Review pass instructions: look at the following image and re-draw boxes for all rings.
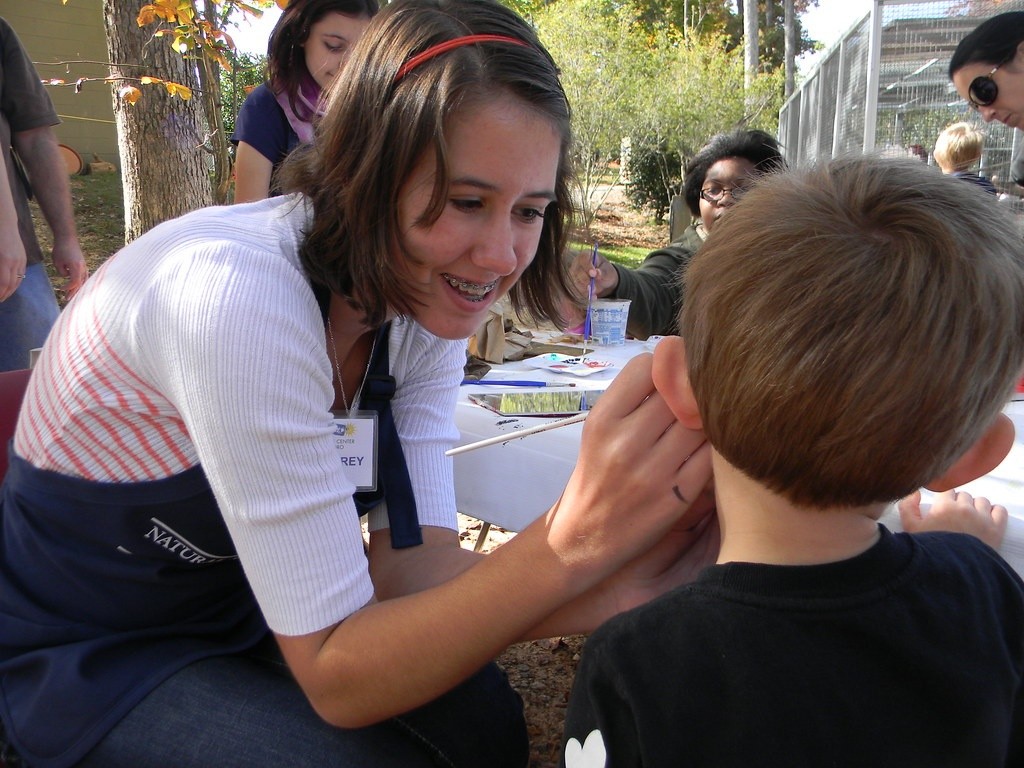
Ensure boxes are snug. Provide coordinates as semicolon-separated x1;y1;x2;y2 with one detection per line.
17;274;26;279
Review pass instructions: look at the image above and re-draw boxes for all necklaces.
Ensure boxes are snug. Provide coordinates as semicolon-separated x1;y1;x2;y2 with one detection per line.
326;317;379;418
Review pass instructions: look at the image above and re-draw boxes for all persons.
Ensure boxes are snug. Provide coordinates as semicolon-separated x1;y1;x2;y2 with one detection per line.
565;128;790;341
0;0;724;768
557;150;1024;768
933;122;997;198
0;16;89;372
229;0;379;205
0;144;27;304
947;11;1024;133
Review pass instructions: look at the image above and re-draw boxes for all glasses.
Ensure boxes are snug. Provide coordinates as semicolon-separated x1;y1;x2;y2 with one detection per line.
700;188;749;200
967;49;1015;112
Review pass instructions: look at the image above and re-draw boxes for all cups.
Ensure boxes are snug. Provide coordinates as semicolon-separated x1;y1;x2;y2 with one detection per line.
591;298;631;345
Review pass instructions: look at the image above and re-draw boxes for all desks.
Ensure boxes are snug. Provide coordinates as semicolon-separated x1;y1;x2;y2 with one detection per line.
453;328;662;553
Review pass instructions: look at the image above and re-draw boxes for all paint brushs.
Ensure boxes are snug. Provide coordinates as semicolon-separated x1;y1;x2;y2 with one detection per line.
445;411;591;456
459;378;580;389
582;242;600;356
